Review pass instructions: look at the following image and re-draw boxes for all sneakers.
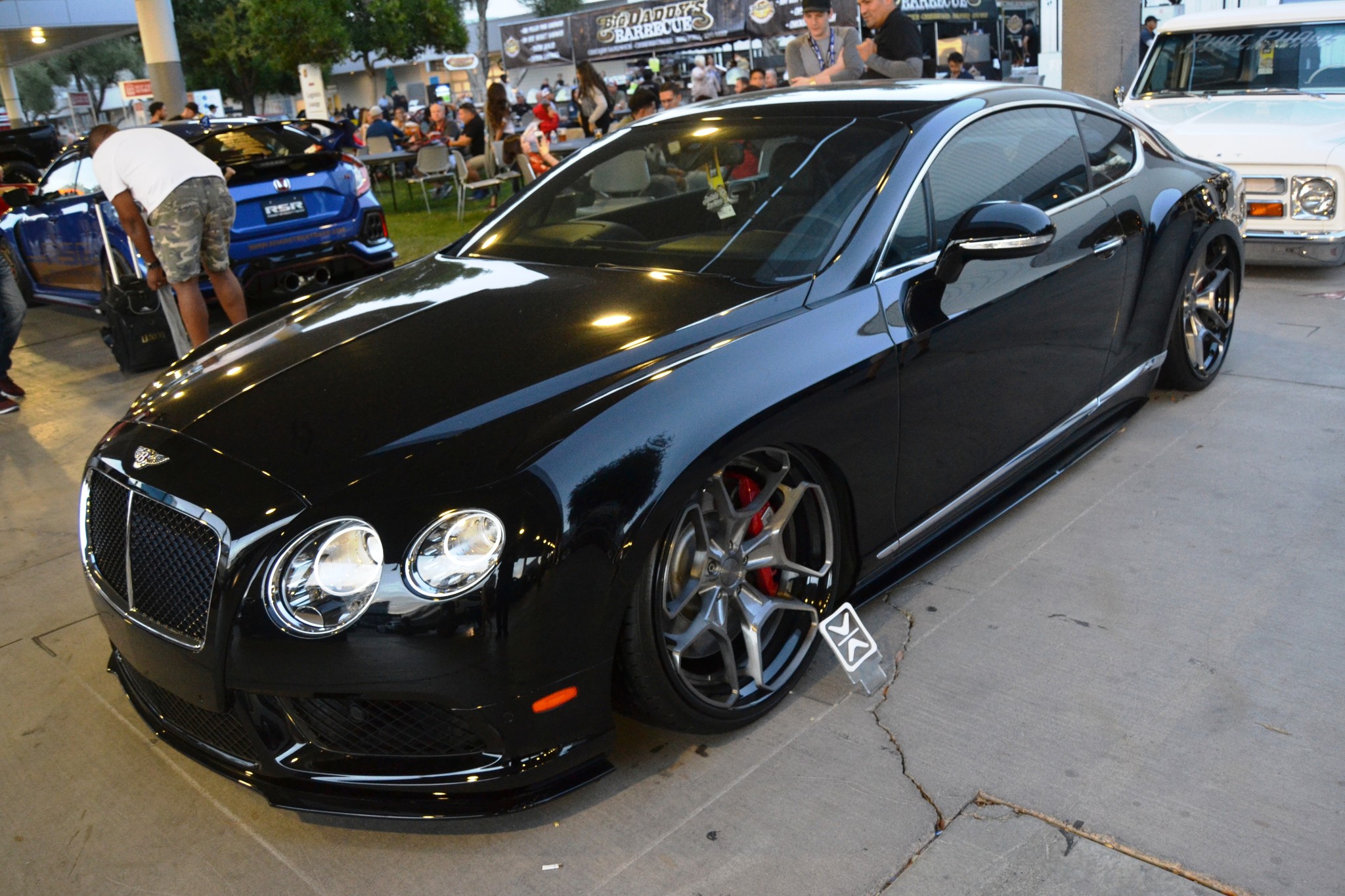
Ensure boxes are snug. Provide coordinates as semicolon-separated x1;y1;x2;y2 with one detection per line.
0;394;19;414
0;375;25;396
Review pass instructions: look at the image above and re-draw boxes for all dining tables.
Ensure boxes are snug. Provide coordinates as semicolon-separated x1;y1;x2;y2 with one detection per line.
543;135;602;156
355;144;459;212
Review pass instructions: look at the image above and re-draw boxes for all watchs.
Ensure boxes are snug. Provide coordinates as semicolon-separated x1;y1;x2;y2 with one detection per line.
144;258;161;271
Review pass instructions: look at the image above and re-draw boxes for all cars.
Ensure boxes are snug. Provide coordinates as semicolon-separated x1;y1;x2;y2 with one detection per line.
73;89;1246;821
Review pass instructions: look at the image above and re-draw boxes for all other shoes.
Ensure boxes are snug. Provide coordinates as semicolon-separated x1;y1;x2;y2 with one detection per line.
434;188;443;199
469;188;489;201
483;203;497;213
442;184;453;199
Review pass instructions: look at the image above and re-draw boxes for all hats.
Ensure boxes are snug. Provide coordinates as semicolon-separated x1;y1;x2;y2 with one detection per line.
185;102;200;114
370;105;382;117
541;87;553;98
209;104;217;110
516;89;525;98
1145;16;1159;22
802;0;831;13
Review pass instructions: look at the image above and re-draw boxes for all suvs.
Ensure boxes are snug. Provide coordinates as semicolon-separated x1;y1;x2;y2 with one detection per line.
1113;0;1344;268
0;114;398;371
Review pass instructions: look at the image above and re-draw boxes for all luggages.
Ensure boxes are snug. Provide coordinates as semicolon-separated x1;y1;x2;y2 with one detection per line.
94;201;178;373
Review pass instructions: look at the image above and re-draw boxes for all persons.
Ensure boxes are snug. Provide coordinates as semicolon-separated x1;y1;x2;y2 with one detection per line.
1022;18;1041;67
55;130;80;155
1139;15;1161;66
206;104;220;119
943;51;974;80
784;0;864;88
297;49;788;213
0;226;28;414
167;102;201;122
0;160;43;184
855;0;927;79
86;123;248;350
147;101;169;125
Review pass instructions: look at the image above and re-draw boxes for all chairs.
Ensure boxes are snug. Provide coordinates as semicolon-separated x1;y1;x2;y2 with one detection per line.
491;142;522;182
508;156;580;225
451;150;510;223
742;141;831;231
577;150;654;216
368;135;402;194
404;144;460;215
